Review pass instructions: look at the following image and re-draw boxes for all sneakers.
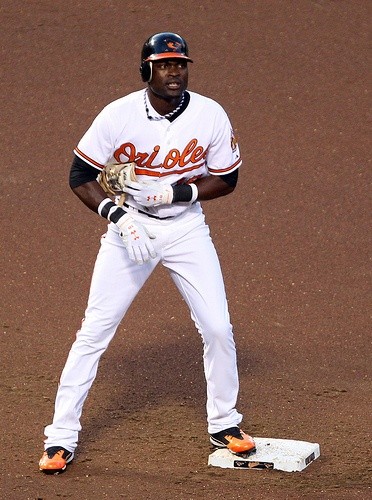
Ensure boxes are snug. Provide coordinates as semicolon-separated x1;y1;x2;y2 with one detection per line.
38;446;75;474
210;427;256;454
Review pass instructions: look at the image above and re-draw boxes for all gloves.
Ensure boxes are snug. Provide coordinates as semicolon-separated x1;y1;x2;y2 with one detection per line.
115;212;157;263
123;179;174;207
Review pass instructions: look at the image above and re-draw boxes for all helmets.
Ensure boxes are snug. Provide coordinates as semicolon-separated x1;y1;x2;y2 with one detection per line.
140;31;194;83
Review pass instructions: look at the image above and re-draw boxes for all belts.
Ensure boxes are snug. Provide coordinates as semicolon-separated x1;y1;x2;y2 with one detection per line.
118;199;174;220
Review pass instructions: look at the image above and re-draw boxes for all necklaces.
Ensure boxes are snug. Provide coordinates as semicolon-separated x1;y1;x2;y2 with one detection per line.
144;87;185;121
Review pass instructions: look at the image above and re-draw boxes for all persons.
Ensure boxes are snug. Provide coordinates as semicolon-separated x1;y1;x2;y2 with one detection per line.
39;31;256;475
